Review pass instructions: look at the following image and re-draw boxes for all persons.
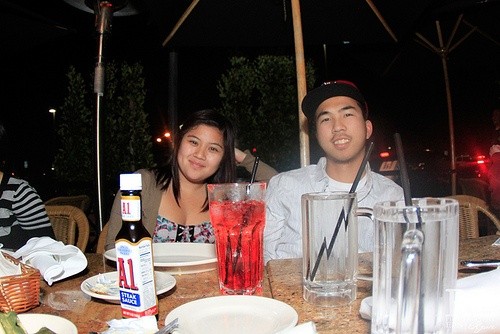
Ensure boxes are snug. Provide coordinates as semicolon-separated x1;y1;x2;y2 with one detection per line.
0;169;56;253
490;109;500;170
104;109;278;252
261;80;406;266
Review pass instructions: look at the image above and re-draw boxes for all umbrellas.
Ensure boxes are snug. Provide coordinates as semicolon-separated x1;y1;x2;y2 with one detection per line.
360;0;500;198
133;0;434;169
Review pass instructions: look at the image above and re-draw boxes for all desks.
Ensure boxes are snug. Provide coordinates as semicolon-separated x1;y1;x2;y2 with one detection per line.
0;235;500;334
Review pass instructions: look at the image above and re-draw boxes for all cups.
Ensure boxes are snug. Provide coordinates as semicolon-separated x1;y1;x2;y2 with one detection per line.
301;191;373;307
370;197;459;334
207;184;267;295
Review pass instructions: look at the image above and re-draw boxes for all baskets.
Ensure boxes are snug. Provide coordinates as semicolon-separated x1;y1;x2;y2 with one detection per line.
0;251;41;314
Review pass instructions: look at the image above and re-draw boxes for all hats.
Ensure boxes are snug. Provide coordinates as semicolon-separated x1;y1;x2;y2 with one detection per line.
301;80;368;120
489;144;500;157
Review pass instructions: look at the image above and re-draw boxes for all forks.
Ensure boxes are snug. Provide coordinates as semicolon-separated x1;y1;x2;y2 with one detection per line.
153;317;179;334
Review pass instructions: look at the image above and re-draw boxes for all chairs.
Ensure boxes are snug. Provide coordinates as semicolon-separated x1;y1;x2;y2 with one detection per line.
46;206;89;253
447;195;500;239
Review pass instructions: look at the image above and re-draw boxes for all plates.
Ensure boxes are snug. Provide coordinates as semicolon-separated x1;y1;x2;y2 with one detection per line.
104;241;219;274
80;271;176;304
165;295;298;334
15;314;78;334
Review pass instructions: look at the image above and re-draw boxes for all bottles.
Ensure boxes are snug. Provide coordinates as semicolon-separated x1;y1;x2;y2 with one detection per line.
115;174;159;322
